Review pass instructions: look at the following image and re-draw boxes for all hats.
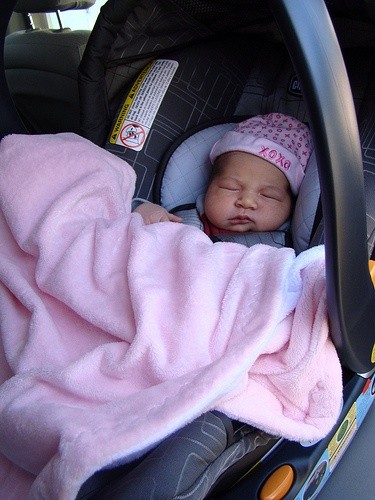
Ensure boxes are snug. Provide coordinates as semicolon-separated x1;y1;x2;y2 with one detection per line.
210;113;313;197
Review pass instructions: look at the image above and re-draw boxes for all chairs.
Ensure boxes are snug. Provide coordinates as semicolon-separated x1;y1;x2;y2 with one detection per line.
4;0;90;134
0;0;375;500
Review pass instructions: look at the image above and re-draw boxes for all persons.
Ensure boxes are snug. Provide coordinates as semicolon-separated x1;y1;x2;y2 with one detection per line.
130;113;315;434
